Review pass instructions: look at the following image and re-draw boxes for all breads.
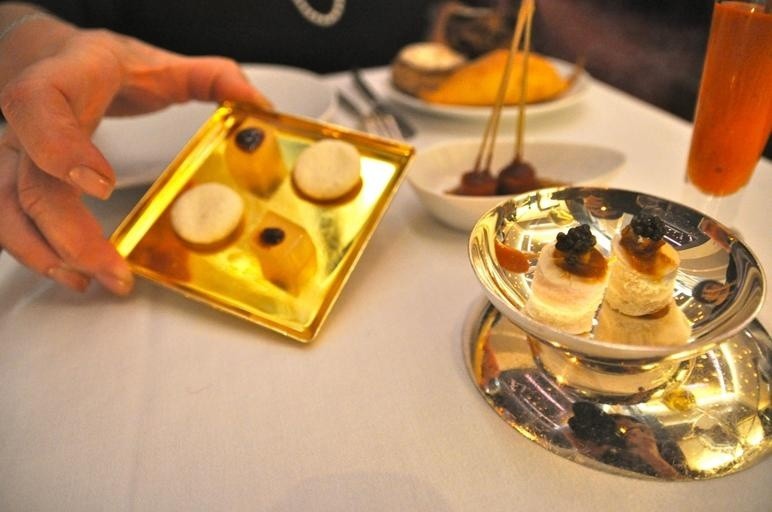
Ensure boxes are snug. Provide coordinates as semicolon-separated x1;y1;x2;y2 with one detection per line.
421;49;574;108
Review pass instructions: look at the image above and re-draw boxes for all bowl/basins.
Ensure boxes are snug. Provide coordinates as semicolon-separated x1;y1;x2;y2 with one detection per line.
406;136;630;233
469;185;767;368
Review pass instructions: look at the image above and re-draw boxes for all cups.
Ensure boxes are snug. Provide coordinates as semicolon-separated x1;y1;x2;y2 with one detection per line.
680;1;772;229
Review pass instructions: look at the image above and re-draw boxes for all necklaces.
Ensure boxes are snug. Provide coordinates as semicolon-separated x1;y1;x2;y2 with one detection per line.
293;0;346;28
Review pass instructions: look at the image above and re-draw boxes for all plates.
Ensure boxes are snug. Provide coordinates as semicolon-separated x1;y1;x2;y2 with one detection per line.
381;55;592;118
90;62;336;190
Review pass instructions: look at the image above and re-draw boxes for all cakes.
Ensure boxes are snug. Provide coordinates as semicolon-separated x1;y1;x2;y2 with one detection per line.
392;39;463;96
170;115;364;296
533;214;679;334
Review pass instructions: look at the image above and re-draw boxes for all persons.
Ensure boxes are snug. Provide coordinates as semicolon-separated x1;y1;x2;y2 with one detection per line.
1;0;693;297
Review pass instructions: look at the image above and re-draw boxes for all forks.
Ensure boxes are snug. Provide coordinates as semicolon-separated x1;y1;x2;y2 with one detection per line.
336;87;401;143
